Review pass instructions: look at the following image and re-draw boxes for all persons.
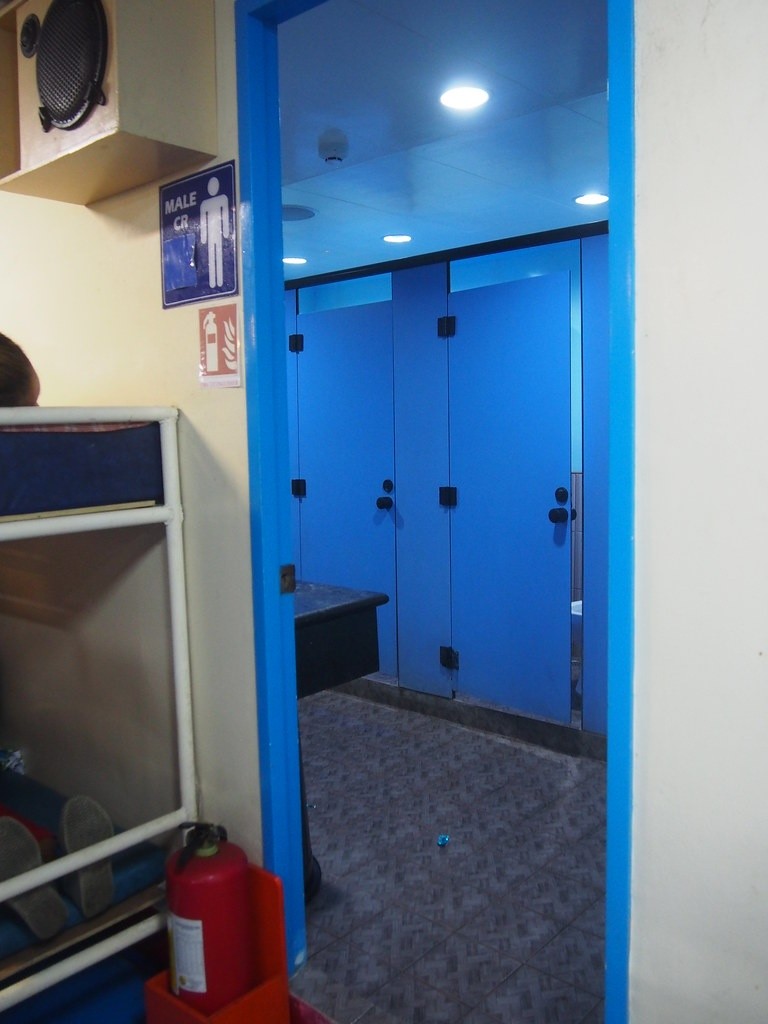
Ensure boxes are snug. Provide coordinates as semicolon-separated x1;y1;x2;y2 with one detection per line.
0;795;115;945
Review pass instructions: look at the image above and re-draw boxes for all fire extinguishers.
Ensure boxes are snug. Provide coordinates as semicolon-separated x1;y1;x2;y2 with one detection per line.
164;821;260;1018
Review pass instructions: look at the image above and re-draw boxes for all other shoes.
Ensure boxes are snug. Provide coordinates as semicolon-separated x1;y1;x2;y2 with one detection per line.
58;795;114;918
0;817;67;940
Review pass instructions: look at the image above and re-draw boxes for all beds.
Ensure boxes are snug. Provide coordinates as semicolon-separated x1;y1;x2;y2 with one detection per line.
1;406;199;1023
